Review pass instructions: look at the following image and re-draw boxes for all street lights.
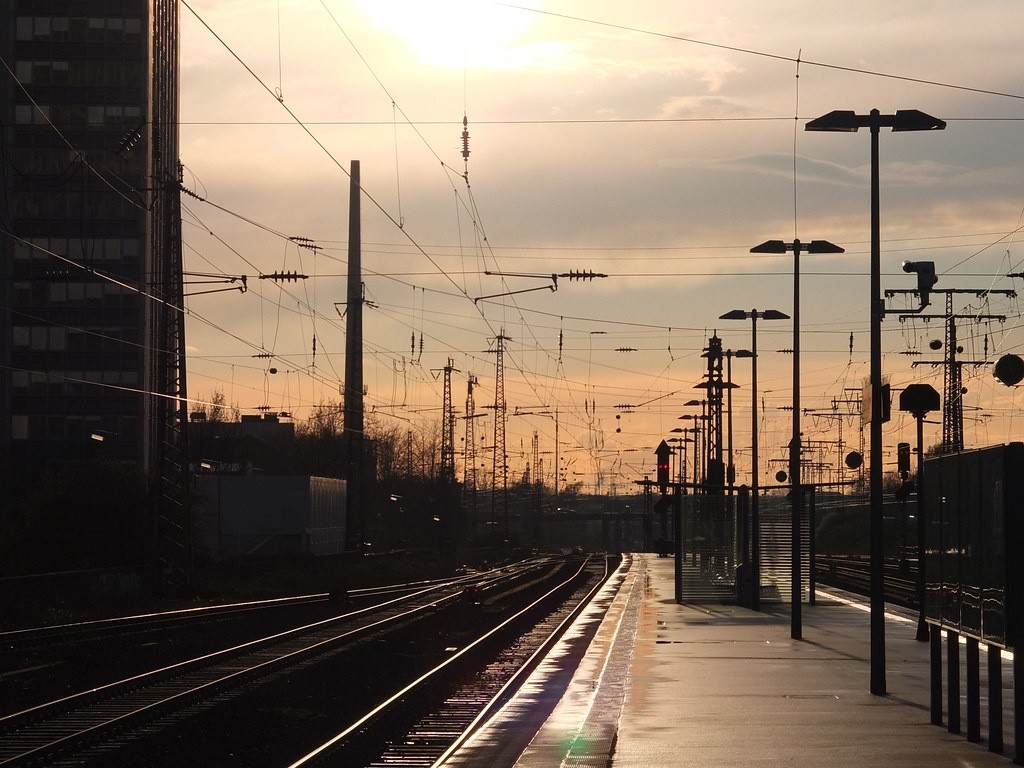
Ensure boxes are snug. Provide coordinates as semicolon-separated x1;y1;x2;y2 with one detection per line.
748;238;847;637
801;106;950;700
667;310;793;607
